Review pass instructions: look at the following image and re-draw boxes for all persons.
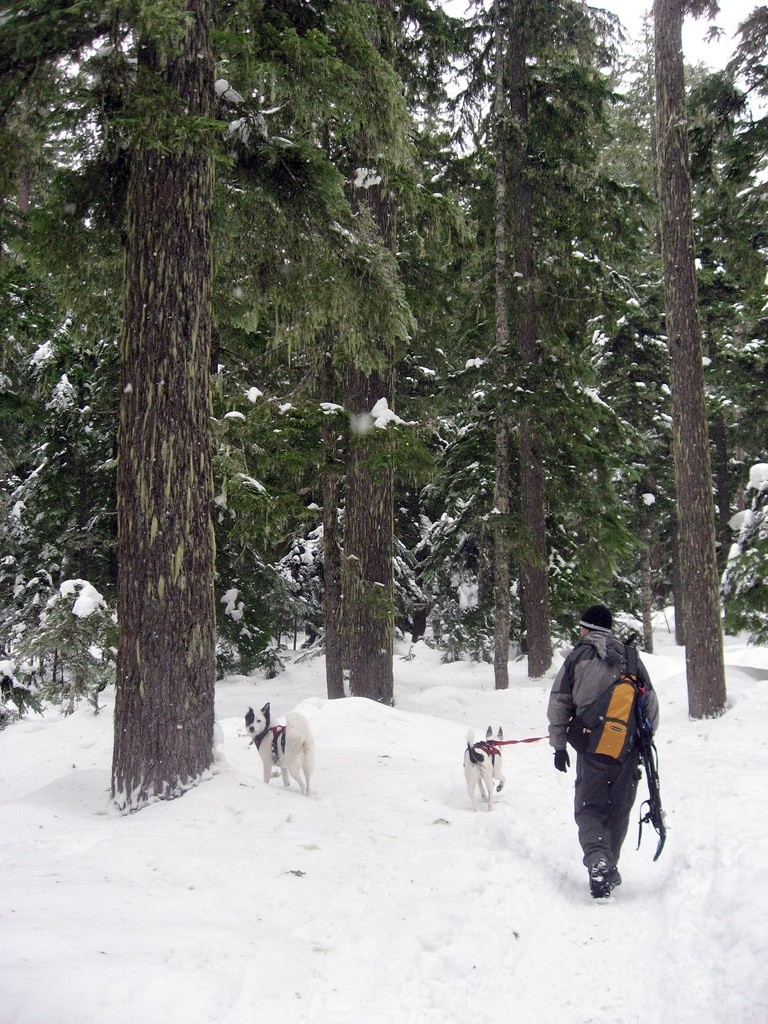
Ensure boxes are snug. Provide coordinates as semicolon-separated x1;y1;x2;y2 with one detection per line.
546;606;659;898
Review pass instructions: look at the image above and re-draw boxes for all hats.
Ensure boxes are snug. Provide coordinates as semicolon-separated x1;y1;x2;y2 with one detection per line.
579;605;613;633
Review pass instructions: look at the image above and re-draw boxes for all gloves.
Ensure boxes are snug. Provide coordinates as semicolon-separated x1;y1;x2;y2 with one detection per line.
554;750;570;772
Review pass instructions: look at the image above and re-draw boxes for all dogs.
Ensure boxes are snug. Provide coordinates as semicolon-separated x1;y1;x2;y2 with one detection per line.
462;725;506;813
243;702;314;797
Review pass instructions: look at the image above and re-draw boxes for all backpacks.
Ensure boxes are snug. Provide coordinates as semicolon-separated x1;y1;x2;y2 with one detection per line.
566;634;653;766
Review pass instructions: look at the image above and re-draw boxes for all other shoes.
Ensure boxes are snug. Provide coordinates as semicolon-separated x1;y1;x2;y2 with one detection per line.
591;861;621;898
